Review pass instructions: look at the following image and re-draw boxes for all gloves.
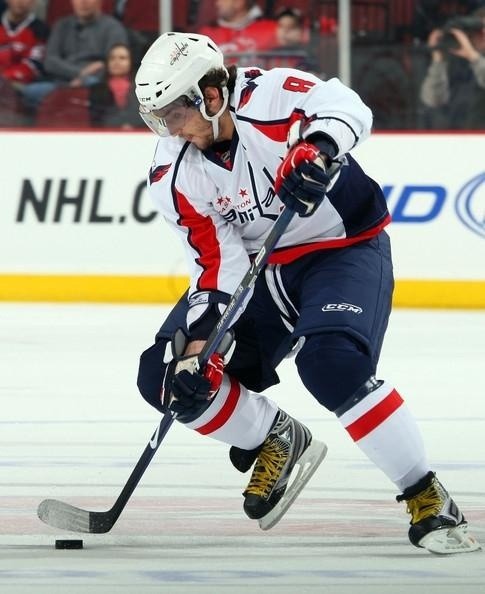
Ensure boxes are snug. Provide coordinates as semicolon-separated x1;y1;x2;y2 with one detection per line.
275;142;331;217
161;327;235;417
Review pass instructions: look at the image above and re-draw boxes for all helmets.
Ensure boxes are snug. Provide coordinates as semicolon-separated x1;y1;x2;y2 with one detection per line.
134;31;230;138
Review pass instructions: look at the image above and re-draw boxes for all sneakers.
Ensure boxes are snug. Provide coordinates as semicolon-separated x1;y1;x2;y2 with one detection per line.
394;471;467;548
230;407;311;519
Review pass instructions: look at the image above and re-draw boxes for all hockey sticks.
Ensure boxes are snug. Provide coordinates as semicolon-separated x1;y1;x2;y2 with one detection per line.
37;205;296;533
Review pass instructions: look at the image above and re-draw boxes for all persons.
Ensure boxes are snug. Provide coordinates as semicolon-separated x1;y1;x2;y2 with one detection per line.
135;32;467;548
1;0;485;130
